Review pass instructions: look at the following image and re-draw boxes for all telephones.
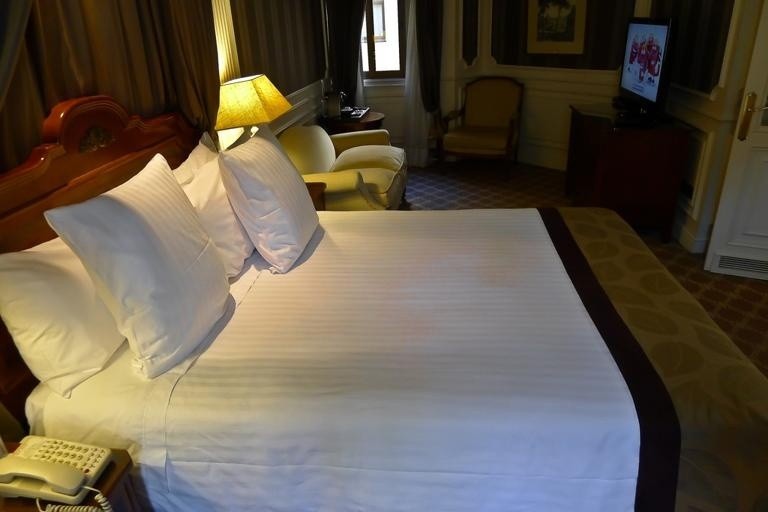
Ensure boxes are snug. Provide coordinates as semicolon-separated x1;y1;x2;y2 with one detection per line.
0;435;114;506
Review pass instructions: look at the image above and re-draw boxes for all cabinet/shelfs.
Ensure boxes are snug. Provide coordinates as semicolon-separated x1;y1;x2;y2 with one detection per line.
565;97;694;244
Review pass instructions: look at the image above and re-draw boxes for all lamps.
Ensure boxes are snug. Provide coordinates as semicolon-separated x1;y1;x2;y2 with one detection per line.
215;72;296;140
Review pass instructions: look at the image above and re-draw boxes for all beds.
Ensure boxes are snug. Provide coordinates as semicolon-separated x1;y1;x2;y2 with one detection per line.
0;84;766;512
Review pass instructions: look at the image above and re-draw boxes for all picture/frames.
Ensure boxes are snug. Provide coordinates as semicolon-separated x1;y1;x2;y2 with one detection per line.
515;0;594;68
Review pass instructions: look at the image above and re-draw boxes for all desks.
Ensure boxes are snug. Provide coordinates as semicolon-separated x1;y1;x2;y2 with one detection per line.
314;105;387;135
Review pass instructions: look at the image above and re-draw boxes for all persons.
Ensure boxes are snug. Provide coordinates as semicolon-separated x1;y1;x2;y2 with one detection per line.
627;30;662;88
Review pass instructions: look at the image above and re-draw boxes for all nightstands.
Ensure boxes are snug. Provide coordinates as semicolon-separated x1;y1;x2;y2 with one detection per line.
1;438;144;512
300;176;327;210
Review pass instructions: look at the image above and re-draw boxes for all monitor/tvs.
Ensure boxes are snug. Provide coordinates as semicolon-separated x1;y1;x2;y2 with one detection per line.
611;16;674;129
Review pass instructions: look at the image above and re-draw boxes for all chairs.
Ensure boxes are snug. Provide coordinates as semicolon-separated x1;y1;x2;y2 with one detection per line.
430;75;526;187
274;121;412;211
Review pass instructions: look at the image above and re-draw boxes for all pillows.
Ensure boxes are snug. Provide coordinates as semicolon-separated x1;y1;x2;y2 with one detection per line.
221;122;321;274
0;233;130;403
169;128;258;285
39;150;235;381
